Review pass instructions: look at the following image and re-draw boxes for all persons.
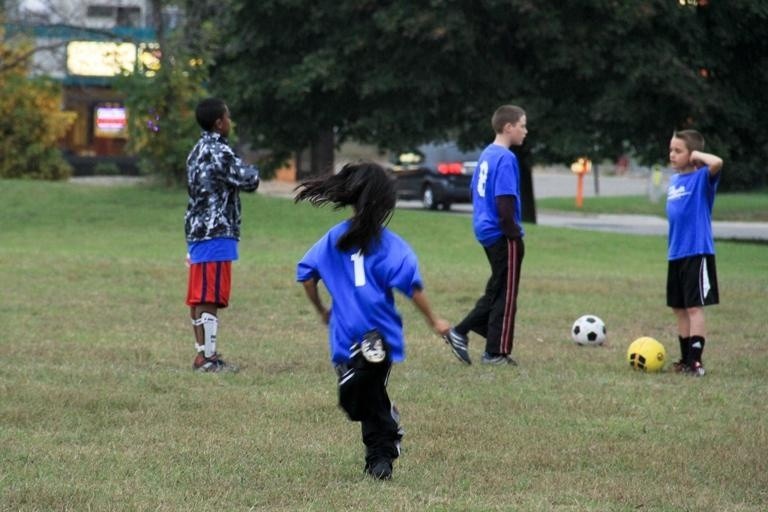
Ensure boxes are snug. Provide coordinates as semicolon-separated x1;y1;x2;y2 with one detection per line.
442;104;529;367
663;129;724;376
291;161;451;479
183;97;260;374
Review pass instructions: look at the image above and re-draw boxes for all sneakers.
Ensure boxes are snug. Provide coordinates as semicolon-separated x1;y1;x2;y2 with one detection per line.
669;359;706;379
360;331;388;367
364;454;394;478
442;326;473;366
193;352;239;374
481;350;518;367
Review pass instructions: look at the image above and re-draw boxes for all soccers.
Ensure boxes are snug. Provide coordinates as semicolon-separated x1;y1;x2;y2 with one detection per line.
571;314;606;346
626;336;666;374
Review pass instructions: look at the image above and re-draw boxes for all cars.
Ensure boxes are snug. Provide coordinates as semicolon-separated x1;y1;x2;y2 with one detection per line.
385;137;488;211
